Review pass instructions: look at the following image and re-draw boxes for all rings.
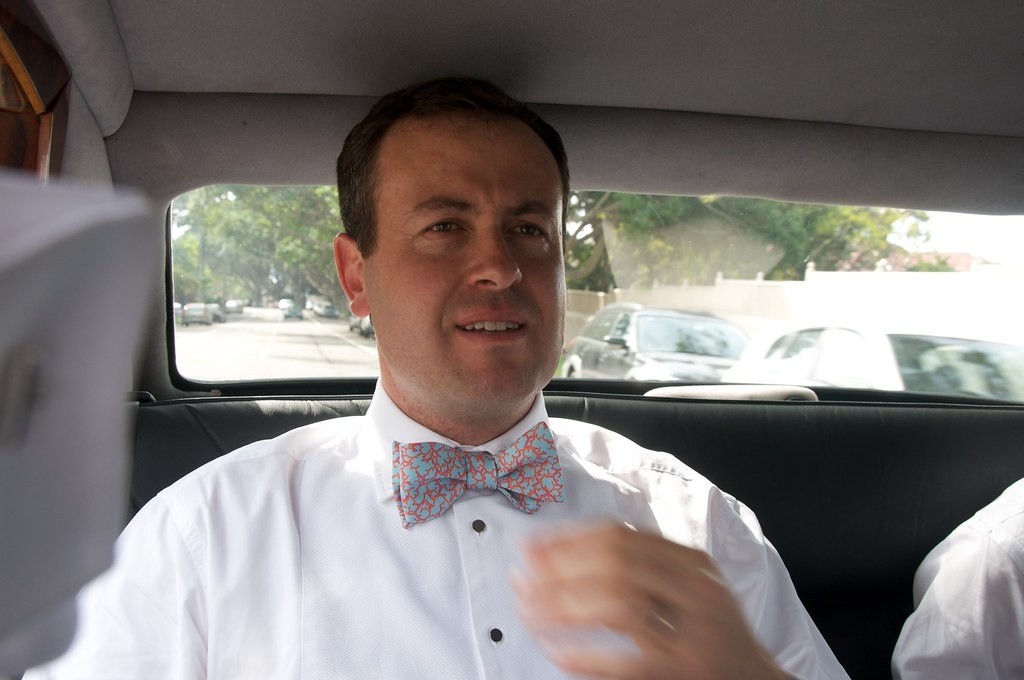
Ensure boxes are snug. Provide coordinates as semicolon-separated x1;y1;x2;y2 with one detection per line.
647;598;669;618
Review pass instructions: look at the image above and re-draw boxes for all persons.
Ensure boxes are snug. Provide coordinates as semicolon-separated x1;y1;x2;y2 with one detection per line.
892;476;1024;680
23;73;854;680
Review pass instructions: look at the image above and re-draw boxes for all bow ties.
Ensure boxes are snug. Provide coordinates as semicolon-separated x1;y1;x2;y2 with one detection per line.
392;422;565;528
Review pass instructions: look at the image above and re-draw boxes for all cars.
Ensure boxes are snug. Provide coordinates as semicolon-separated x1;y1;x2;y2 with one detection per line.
226;297;251;315
182;302;214;326
207;303;227;323
561;300;781;381
279;296;374;339
738;315;1024;403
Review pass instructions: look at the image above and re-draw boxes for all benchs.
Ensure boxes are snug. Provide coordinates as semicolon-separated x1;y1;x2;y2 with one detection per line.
131;391;1024;680
